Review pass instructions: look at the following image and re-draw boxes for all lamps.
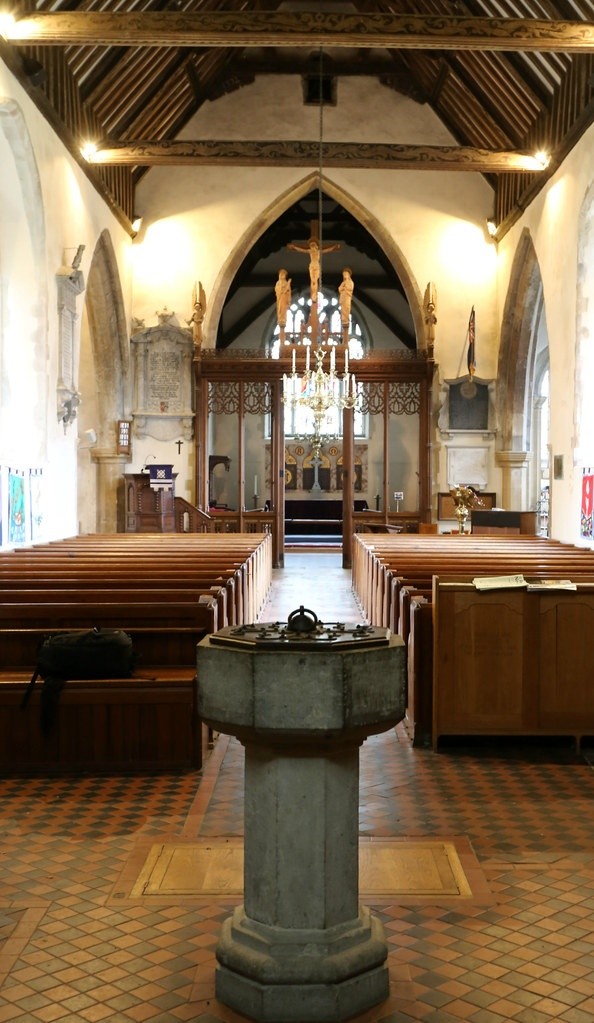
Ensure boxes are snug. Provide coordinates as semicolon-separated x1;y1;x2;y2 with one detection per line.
279;50;360;459
130;214;142;233
485;216;498;233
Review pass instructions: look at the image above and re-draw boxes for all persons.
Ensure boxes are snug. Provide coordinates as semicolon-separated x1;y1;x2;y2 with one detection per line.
275;270;292;323
339;268;354;324
290;242;337;288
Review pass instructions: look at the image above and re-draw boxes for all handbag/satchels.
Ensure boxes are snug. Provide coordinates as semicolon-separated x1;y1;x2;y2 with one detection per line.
22;627;132;705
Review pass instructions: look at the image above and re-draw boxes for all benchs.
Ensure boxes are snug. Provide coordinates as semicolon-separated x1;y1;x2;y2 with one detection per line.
0;530;273;776
350;531;594;757
